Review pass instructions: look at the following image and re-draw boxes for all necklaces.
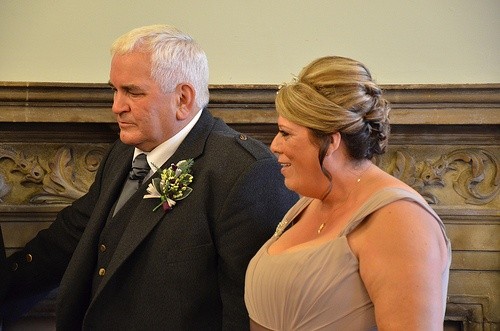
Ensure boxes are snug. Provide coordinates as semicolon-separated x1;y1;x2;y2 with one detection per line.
317;163;371;233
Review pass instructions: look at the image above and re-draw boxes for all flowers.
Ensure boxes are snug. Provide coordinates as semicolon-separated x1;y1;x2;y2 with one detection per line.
140;158;195;214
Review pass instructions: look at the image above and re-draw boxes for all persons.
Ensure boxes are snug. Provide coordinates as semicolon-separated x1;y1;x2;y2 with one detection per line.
0;24;299;331
244;56;453;331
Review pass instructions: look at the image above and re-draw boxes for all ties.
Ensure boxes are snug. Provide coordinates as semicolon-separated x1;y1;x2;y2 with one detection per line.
113;156;150;216
